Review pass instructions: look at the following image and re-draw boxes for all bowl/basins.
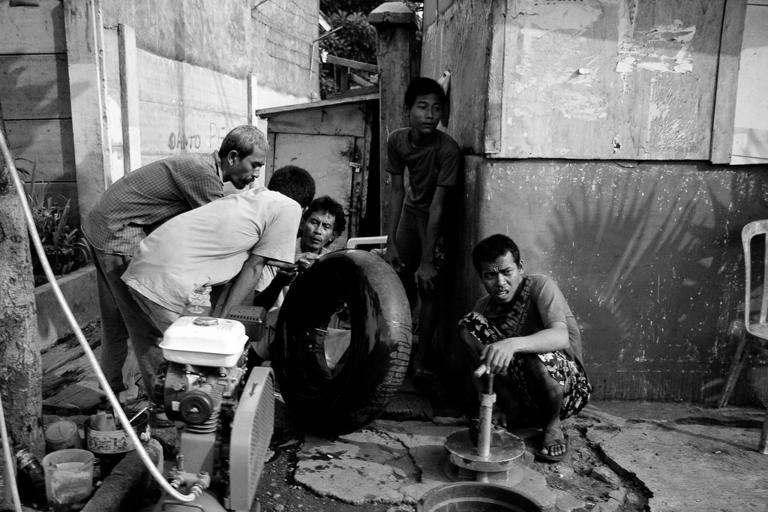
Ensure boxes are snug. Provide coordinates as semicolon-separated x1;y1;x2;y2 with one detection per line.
82;411;152;456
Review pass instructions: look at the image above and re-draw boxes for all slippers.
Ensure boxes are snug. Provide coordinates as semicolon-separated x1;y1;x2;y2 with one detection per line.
531;429;569;464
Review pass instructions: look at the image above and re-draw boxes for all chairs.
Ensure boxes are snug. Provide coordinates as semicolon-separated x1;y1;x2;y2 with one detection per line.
720;217;768;455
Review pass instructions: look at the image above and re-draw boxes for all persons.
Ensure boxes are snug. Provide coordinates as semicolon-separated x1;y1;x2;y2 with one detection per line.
457;234;594;463
80;124;346;407
382;76;468;383
